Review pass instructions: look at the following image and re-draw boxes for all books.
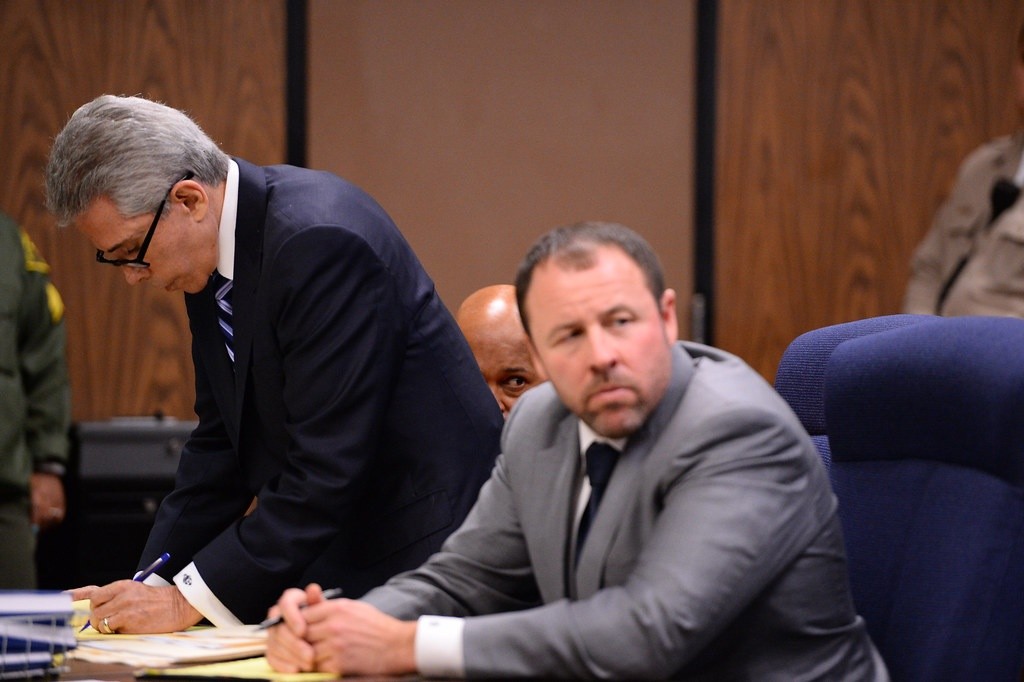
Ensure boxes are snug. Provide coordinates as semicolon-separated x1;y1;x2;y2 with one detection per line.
64;624;268;667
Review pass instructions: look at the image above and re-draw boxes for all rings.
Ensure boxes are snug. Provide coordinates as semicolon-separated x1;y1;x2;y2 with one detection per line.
103;618;114;633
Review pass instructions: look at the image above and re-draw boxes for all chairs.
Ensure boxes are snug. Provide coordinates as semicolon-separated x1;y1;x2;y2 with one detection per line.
776;312;1024;682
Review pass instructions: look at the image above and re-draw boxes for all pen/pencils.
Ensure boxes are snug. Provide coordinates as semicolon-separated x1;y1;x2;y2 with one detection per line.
76;553;171;634
249;587;343;635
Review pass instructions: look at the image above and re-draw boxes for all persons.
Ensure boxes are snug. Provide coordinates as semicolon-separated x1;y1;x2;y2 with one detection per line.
40;94;505;634
454;284;549;423
905;24;1024;319
266;219;888;682
0;214;70;590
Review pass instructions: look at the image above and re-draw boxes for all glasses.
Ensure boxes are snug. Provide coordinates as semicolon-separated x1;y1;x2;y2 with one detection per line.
96;170;195;270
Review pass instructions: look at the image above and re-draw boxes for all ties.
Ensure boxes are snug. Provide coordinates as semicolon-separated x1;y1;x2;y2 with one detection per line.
571;442;624;570
214;269;236;366
